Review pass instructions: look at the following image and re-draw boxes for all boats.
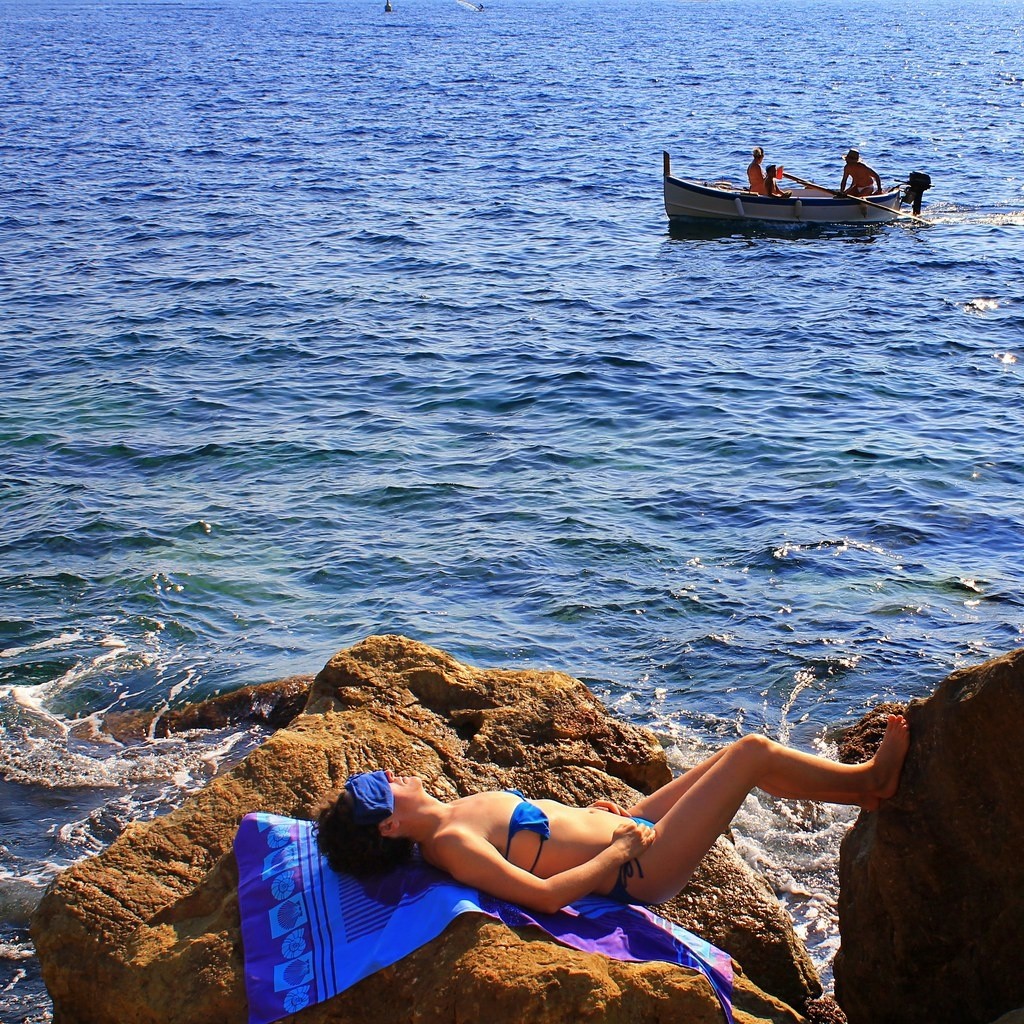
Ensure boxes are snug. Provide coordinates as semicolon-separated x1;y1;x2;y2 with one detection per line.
662;150;902;232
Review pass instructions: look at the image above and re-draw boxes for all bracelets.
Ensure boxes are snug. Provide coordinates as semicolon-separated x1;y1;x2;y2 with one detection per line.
878;188;882;191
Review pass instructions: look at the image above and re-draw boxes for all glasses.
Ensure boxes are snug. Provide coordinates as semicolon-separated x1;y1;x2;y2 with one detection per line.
757;155;764;158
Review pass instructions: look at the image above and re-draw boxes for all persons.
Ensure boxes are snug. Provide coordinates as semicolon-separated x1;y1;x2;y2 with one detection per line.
312;714;909;916
747;147;765;194
833;149;882;197
763;164;793;197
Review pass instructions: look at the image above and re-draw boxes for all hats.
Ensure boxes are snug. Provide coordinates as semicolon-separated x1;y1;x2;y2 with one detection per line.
844;150;859;162
766;164;776;170
